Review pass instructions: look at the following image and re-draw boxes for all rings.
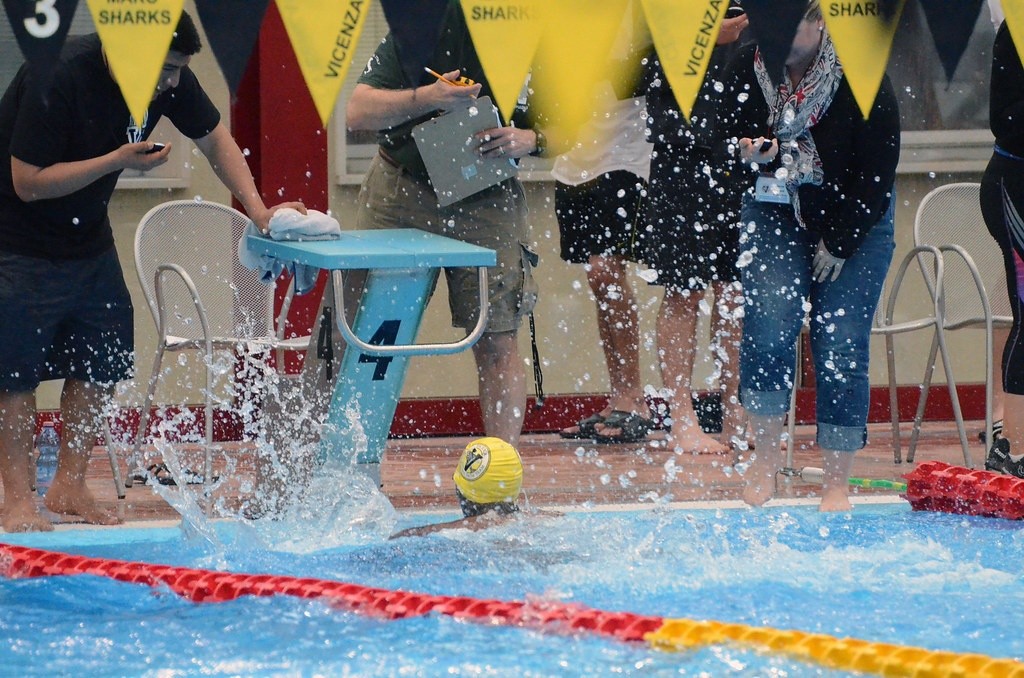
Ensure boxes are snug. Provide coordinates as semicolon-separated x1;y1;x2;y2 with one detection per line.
499;146;504;154
824;265;833;269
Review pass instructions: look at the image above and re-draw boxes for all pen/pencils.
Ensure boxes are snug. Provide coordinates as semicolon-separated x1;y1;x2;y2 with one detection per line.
424;67;477;100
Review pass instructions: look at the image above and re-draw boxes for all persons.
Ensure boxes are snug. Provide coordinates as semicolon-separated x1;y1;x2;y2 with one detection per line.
388;437;565;542
0;9;306;533
713;0;901;511
346;0;576;488
550;0;790;456
979;19;1024;483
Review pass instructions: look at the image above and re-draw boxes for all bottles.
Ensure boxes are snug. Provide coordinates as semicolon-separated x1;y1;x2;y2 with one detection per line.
35;420;60;495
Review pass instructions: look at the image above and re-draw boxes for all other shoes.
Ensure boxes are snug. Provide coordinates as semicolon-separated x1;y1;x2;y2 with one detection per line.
979;419;1003;444
985;434;1010;472
1001;454;1024;479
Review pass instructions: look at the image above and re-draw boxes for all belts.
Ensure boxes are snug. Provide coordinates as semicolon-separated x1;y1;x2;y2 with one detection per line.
377;147;433;192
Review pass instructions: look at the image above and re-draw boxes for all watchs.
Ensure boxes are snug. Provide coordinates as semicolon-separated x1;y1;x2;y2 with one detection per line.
528;125;547;159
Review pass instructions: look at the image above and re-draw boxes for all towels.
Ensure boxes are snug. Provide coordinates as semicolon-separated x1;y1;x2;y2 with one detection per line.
236;206;341;295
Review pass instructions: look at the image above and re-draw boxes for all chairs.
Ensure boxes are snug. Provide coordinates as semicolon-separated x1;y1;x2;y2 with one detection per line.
786;246;972;481
125;200;298;520
906;182;1012;469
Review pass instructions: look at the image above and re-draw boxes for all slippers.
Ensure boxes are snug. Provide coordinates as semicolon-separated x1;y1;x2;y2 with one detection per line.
593;408;656;444
134;463;218;485
559;413;605;439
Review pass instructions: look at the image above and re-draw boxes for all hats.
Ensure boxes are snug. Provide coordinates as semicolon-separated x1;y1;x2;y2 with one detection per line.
453;437;523;503
